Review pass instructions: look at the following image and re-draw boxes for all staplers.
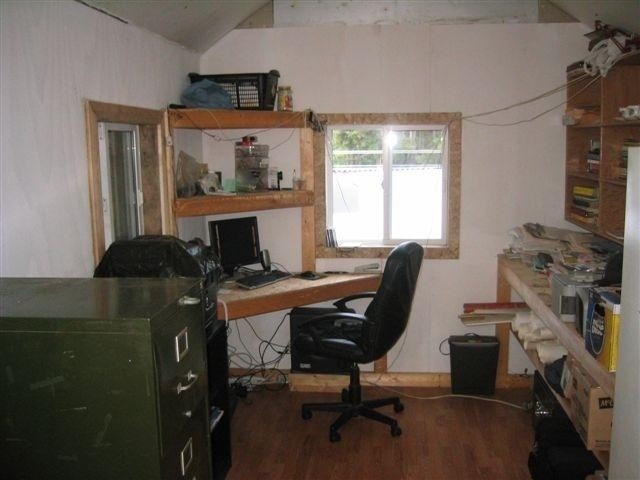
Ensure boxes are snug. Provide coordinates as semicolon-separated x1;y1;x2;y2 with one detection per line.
354;263;382;274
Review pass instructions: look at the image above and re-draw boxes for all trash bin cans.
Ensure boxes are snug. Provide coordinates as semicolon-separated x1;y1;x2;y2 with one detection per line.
448;335;500;395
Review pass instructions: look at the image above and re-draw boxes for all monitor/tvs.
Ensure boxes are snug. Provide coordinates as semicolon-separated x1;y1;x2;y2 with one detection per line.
208;217;260;276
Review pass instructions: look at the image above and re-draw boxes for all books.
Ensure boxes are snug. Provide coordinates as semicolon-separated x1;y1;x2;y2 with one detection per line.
211;410;225;431
562;100;601;225
211;406;219;424
604;229;625;241
507;221;620;292
617;138;640;180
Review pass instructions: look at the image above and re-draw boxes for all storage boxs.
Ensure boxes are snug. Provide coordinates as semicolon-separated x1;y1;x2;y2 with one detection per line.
560;356;614;452
553;274;600;323
585;286;620;374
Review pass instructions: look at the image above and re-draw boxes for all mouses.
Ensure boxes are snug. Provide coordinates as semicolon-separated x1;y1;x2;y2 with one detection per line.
301;270;319;278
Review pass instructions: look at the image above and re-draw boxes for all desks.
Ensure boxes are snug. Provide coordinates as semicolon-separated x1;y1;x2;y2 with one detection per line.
496;253;616;480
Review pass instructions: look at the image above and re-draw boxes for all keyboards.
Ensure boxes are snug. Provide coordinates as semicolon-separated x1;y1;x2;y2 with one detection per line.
236;269;291;290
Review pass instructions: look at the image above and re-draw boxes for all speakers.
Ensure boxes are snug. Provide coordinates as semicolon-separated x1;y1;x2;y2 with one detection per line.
260;249;271;272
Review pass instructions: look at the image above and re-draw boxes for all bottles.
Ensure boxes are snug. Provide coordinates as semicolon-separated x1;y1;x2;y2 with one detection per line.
278;85;292;112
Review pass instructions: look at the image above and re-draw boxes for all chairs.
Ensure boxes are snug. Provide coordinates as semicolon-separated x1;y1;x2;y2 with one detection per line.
290;241;424;444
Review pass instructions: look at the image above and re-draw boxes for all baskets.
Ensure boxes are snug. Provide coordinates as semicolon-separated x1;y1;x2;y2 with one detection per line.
188;70;280;111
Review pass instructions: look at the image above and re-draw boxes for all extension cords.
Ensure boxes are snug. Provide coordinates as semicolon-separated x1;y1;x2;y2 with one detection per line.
228;375;265;387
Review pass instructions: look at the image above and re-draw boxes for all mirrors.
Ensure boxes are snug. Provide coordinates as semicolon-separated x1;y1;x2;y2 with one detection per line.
83;98;166;270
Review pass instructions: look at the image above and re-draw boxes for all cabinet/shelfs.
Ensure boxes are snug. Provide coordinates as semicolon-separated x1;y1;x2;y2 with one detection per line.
0;275;214;480
564;43;640;247
160;107;388;376
